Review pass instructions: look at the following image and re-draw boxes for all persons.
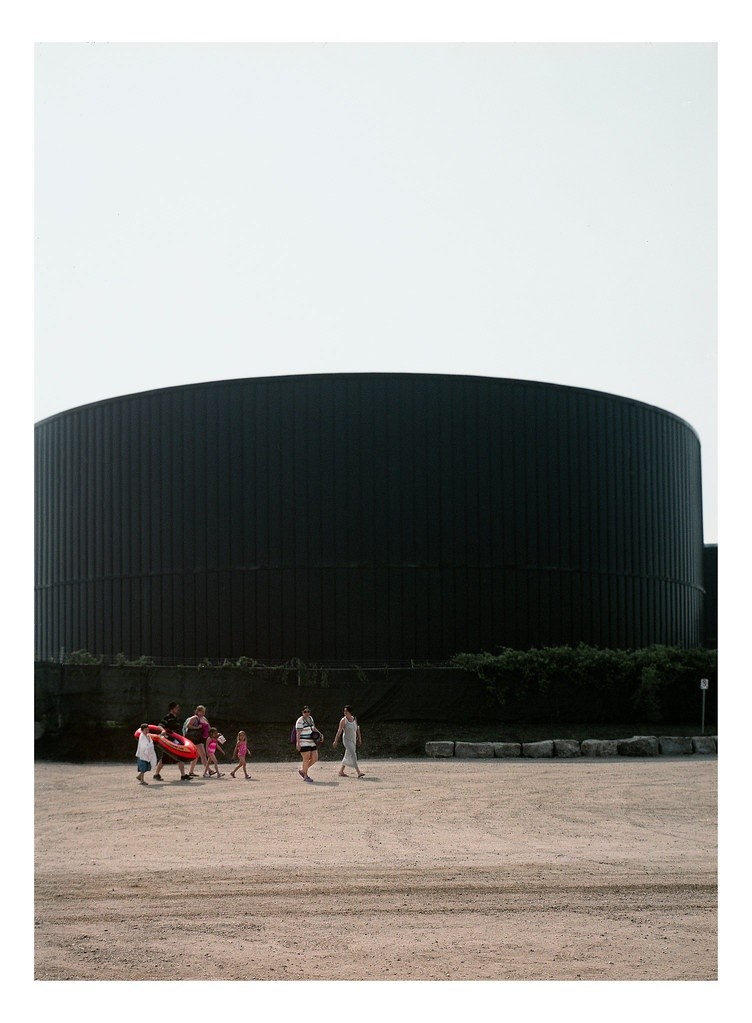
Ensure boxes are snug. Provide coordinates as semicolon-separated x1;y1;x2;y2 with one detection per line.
152;701;193;781
202;727;226;778
229;731;252;779
135;724;155;785
185;705;222;777
333;705;366;777
295;705;324;781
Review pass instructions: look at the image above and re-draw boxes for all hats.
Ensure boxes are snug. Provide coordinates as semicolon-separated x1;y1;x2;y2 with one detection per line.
168;700;178;712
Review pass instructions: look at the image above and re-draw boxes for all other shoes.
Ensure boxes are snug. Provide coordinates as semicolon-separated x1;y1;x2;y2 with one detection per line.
358;773;365;778
153;774;163;781
181;774;193;780
245;775;251;779
230;772;236;778
189;772;199;777
304;776;313;782
298;769;304;777
209;771;216;775
217;773;225;778
136;777;141;781
203;772;210;778
139;782;148;785
339;772;347;776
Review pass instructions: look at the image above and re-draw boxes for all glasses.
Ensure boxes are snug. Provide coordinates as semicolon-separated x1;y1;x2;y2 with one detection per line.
303;711;310;713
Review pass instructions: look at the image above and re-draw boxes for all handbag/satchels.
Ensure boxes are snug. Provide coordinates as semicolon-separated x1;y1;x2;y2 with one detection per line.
290;725;296;744
217;735;226;745
311;731;321;745
184;716;204;745
